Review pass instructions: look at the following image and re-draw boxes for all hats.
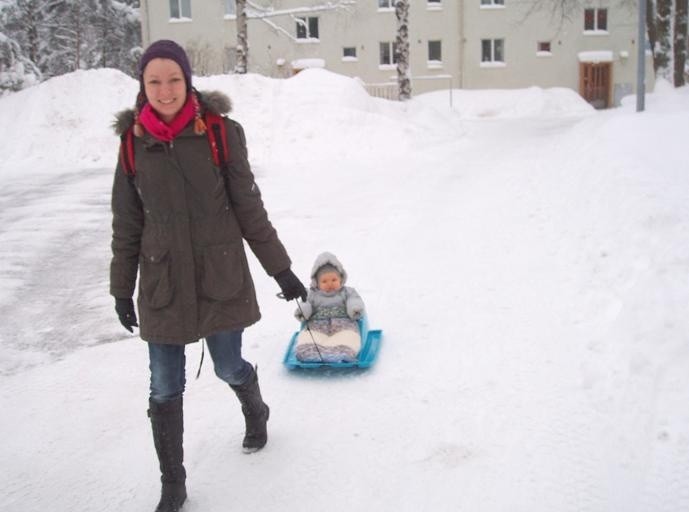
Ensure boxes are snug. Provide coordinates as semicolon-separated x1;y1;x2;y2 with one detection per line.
139;39;192;90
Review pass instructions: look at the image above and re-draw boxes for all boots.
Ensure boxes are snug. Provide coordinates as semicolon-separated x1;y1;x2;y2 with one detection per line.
146;394;190;512
227;361;271;454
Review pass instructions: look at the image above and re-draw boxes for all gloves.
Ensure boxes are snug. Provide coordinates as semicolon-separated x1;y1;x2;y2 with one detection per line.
113;297;139;334
281;285;309;303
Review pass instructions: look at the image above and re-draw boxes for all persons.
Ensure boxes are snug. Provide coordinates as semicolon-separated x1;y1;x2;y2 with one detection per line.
108;38;310;512
293;250;366;366
